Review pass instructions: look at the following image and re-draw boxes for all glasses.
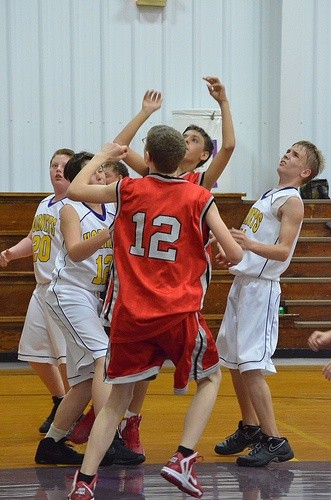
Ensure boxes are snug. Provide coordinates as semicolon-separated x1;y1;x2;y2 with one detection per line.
141;137;148;146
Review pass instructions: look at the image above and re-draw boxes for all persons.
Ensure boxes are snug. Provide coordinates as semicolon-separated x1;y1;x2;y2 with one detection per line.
65;124;243;500
307;329;331;382
214;139;325;468
0;75;236;466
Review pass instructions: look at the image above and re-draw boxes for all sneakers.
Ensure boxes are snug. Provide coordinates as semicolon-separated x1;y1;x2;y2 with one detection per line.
226;465;295;494
68;468;99;500
99;431;146;466
71;405;97;443
35;436;85;465
214;420;262;454
161;452;205;498
237;433;294;466
39;395;64;433
124;468;145;498
115;413;147;455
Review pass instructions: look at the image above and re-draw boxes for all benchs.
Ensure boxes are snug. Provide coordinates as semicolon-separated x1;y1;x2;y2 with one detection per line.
0;192;331;362
